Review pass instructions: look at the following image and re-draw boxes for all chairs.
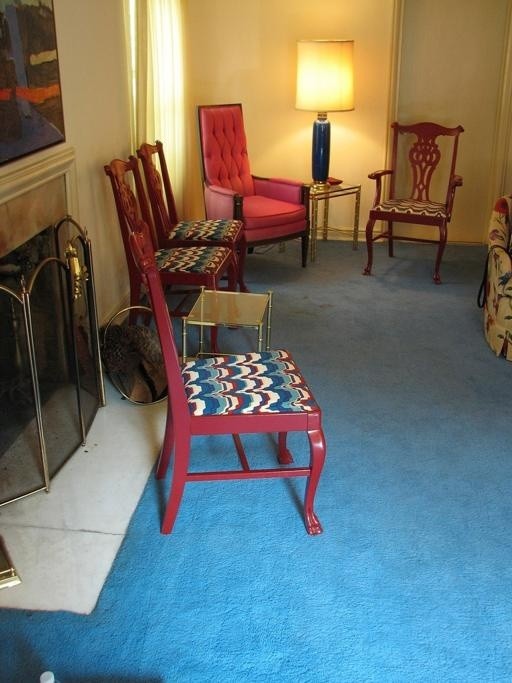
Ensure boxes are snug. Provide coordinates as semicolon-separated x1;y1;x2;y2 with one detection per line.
361;121;465;286
137;140;250;317
103;153;237;354
120;219;328;534
483;194;511;362
197;106;310;275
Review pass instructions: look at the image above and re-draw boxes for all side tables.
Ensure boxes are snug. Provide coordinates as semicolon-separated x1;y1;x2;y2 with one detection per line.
180;285;273;354
278;179;362;262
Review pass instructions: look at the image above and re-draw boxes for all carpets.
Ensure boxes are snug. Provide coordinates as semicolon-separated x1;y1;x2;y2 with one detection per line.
1;236;512;683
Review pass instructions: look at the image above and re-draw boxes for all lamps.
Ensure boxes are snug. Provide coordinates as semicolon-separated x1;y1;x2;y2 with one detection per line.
294;38;357;189
